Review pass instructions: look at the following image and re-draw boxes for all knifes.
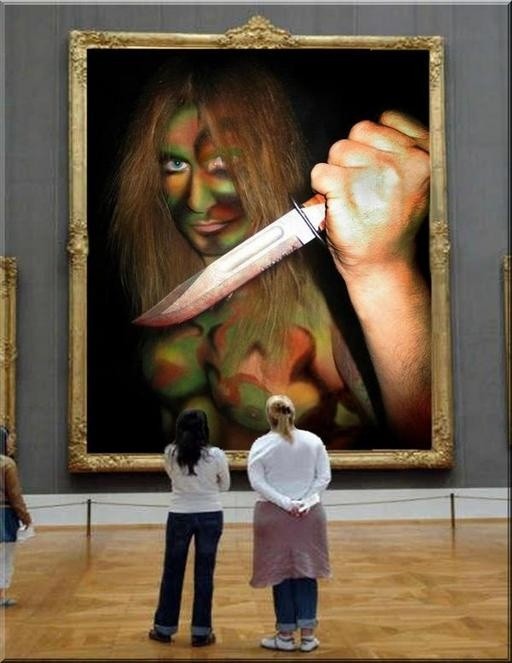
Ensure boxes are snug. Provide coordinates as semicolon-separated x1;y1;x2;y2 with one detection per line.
129;188;325;332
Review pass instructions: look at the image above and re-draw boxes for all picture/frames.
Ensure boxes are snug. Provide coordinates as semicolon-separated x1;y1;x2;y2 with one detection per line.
501;254;512;452
0;256;19;461
65;14;457;475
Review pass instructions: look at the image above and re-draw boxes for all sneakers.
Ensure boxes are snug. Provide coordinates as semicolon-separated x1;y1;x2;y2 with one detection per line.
261;633;296;650
191;633;216;647
300;636;319;651
149;630;170;640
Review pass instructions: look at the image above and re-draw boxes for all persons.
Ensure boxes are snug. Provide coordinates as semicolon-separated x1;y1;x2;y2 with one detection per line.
0;424;36;606
98;68;431;453
142;407;232;647
246;392;335;652
